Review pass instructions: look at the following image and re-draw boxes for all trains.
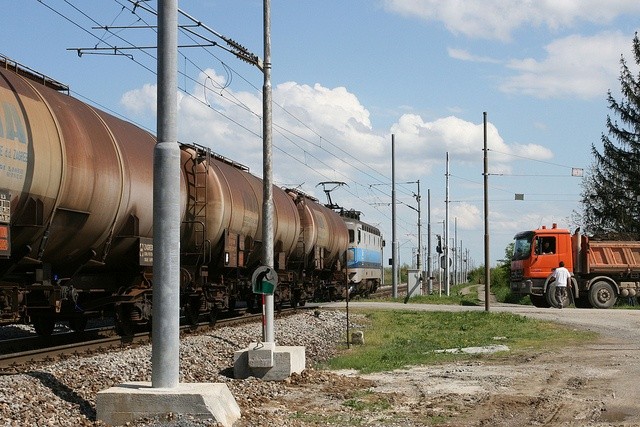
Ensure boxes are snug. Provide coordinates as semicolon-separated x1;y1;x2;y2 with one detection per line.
1;55;385;333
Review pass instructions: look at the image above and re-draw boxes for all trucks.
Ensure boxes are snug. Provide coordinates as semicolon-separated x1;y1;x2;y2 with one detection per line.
511;223;636;308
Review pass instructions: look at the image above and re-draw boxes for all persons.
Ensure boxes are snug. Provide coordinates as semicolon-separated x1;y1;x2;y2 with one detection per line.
542;242;554;254
553;260;573;310
